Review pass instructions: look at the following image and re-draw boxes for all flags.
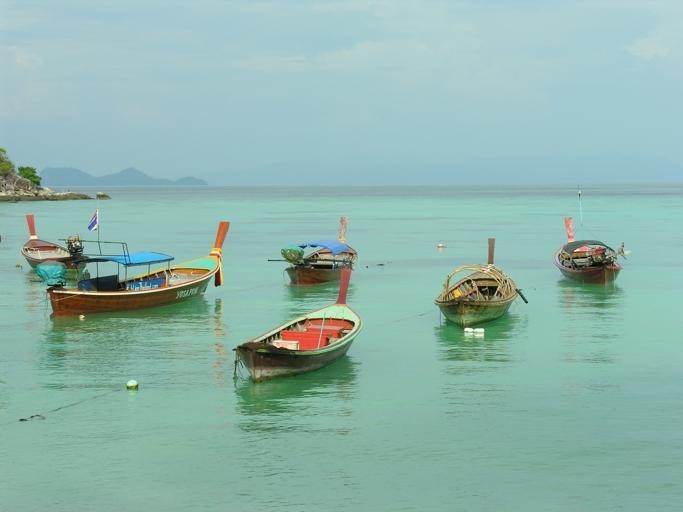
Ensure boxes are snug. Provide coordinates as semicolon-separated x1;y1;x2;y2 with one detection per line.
88;209;98;232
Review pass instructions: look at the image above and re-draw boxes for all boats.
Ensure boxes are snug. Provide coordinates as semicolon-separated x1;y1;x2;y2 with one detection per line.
229;268;367;388
44;218;232;319
434;236;529;328
19;213;89;282
277;213;357;287
553;215;626;285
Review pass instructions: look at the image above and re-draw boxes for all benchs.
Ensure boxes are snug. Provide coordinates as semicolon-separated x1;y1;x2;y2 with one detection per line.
307;322;344;338
